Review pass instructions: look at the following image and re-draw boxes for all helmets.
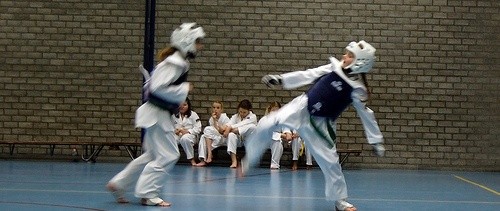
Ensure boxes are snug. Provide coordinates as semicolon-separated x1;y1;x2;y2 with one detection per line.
341;40;376;75
169;22;206;58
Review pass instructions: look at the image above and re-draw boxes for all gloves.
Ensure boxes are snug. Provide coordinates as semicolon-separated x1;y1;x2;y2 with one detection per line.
373;143;386;158
261;73;281;88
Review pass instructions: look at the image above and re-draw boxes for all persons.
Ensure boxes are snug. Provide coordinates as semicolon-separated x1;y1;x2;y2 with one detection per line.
195;100;230;167
240;40;385;211
224;99;257;167
292;130;312;171
106;23;205;207
264;100;292;169
173;97;202;166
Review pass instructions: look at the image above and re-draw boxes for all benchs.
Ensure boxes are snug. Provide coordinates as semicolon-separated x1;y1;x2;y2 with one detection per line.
0;140;361;166
